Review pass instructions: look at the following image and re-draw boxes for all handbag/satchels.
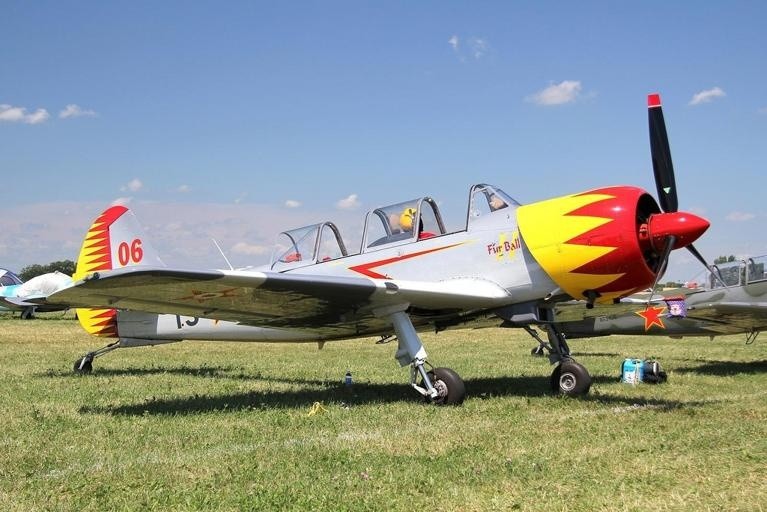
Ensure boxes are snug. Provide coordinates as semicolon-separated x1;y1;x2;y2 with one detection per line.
643;359;667;384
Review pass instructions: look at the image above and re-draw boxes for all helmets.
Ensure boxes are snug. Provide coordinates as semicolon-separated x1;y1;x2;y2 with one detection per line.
399;208;423;232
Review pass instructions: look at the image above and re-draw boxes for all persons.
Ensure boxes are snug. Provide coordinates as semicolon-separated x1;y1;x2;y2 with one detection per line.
398;206;437;241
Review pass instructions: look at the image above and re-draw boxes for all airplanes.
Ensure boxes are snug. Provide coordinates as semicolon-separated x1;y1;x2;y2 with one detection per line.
531;255;766;357
0;268;79;320
20;92;730;407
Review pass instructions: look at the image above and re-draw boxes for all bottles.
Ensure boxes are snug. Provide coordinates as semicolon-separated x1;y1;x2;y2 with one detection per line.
345;368;351;383
634;361;640;383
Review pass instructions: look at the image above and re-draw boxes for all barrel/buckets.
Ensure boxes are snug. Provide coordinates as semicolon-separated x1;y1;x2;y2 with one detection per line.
644;359;659;376
623;357;644;386
